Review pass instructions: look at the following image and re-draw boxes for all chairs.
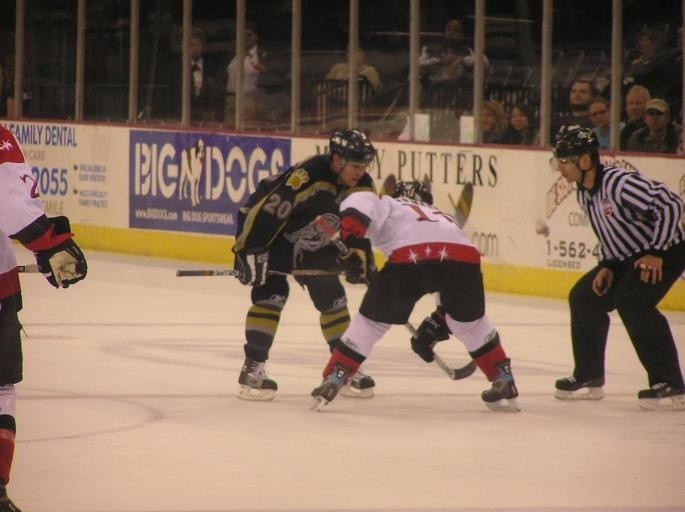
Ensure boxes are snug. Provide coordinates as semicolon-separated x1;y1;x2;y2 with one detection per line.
482;46;632;118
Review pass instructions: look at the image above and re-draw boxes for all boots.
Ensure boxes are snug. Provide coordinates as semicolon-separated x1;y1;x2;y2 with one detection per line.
482;358;518;402
239;357;277;390
352;372;374;389
311;361;349;402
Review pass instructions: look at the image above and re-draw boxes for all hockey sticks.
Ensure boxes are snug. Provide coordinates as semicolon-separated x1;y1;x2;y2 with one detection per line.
315;214;477;380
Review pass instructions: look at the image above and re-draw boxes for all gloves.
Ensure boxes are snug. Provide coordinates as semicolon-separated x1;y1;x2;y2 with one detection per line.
411;309;451;362
234;251;276;286
340;239;372;283
9;214;87;288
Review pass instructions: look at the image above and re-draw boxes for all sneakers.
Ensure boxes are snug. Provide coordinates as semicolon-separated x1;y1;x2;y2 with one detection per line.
557;377;605;390
638;382;685;397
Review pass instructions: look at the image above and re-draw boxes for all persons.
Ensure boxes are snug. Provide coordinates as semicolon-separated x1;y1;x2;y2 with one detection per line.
419;19;494;86
231;128;379;390
1;125;87;512
222;22;283;122
167;31;220;124
482;21;685;154
308;177;520;404
549;126;685;399
324;45;379;102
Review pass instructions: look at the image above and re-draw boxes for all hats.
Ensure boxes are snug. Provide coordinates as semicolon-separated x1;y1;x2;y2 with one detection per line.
645;99;669;113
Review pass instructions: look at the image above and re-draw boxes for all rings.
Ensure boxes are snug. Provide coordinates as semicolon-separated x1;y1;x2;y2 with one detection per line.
646;265;651;270
640;265;645;269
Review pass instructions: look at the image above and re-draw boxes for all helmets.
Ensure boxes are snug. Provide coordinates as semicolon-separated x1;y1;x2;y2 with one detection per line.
554;125;599;158
330;129;374;162
392;181;432;203
